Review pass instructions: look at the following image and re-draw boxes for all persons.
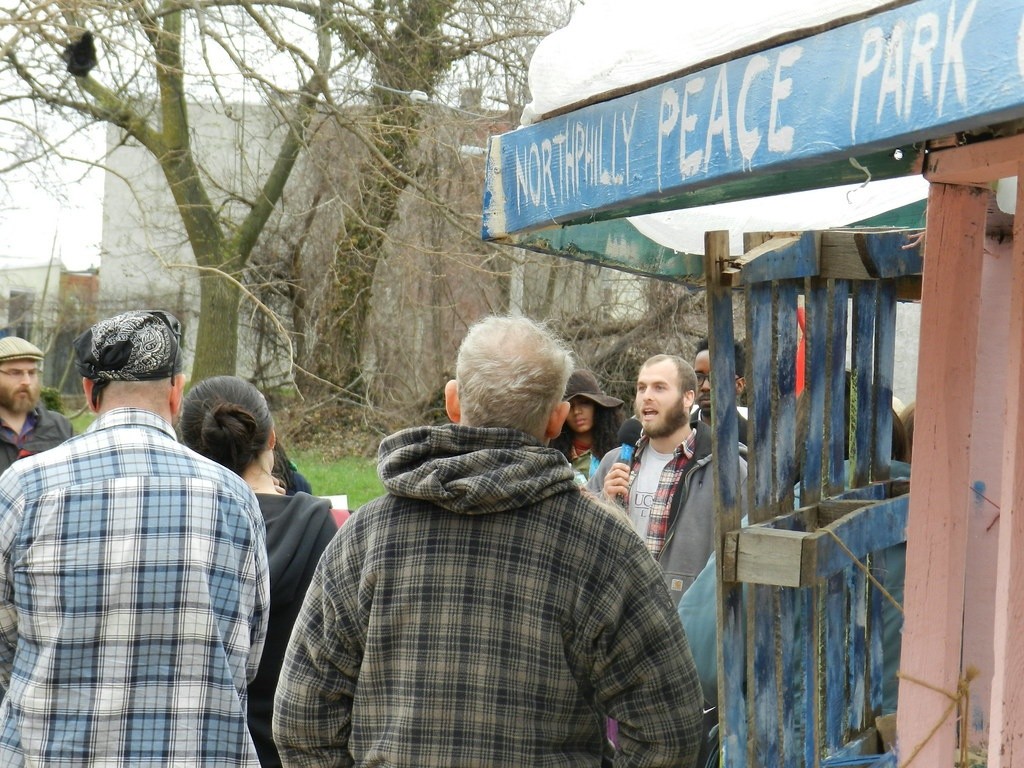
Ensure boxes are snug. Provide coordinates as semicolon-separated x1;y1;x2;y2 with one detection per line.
0;335;76;476
579;354;748;611
271;318;704;766
676;377;917;708
689;338;748;443
0;310;270;768
273;445;313;495
550;369;625;480
180;374;340;768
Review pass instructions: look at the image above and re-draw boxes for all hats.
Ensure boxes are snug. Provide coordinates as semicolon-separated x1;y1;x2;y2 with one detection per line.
561;369;624;416
0;337;45;362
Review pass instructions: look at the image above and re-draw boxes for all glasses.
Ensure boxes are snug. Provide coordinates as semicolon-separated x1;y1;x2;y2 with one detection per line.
693;372;741;388
139;306;183;385
0;369;44;378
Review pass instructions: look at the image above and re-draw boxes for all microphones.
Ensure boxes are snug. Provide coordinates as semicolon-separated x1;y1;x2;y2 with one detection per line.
615;419;644;506
